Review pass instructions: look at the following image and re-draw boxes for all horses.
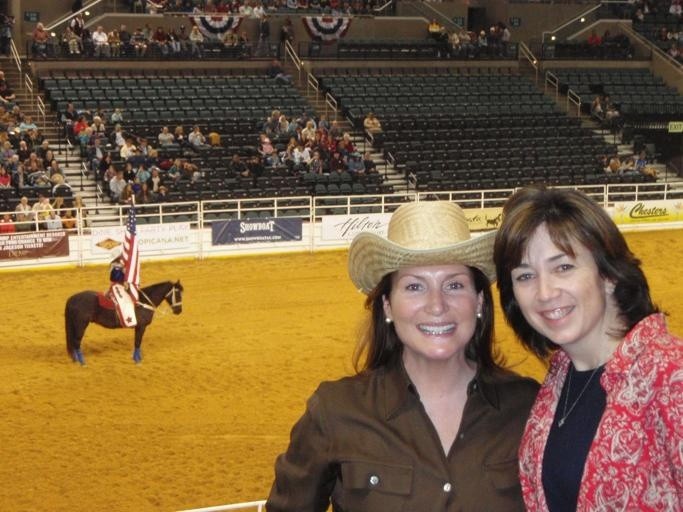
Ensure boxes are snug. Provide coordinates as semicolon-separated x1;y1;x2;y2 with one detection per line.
63;280;183;365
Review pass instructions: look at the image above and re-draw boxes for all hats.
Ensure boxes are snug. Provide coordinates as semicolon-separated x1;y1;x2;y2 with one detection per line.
348;201;500;296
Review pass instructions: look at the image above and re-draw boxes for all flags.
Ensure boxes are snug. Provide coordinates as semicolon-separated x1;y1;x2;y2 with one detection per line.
120;207;140;304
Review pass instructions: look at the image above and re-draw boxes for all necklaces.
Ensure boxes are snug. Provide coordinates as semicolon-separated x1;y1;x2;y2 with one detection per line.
558;362;599;427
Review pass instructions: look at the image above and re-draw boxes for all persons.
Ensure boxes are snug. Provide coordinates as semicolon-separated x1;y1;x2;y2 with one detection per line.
428;18;511;60
0;14;12;57
0;71;86;234
592;94;622;122
110;258;138;327
32;13;295;60
588;0;683;67
602;151;659;181
265;262;541;512
60;103;221;205
493;184;683;512
132;0;390;16
228;110;383;186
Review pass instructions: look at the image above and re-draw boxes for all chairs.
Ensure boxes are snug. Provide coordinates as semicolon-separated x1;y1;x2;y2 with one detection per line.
1;64;683;201
